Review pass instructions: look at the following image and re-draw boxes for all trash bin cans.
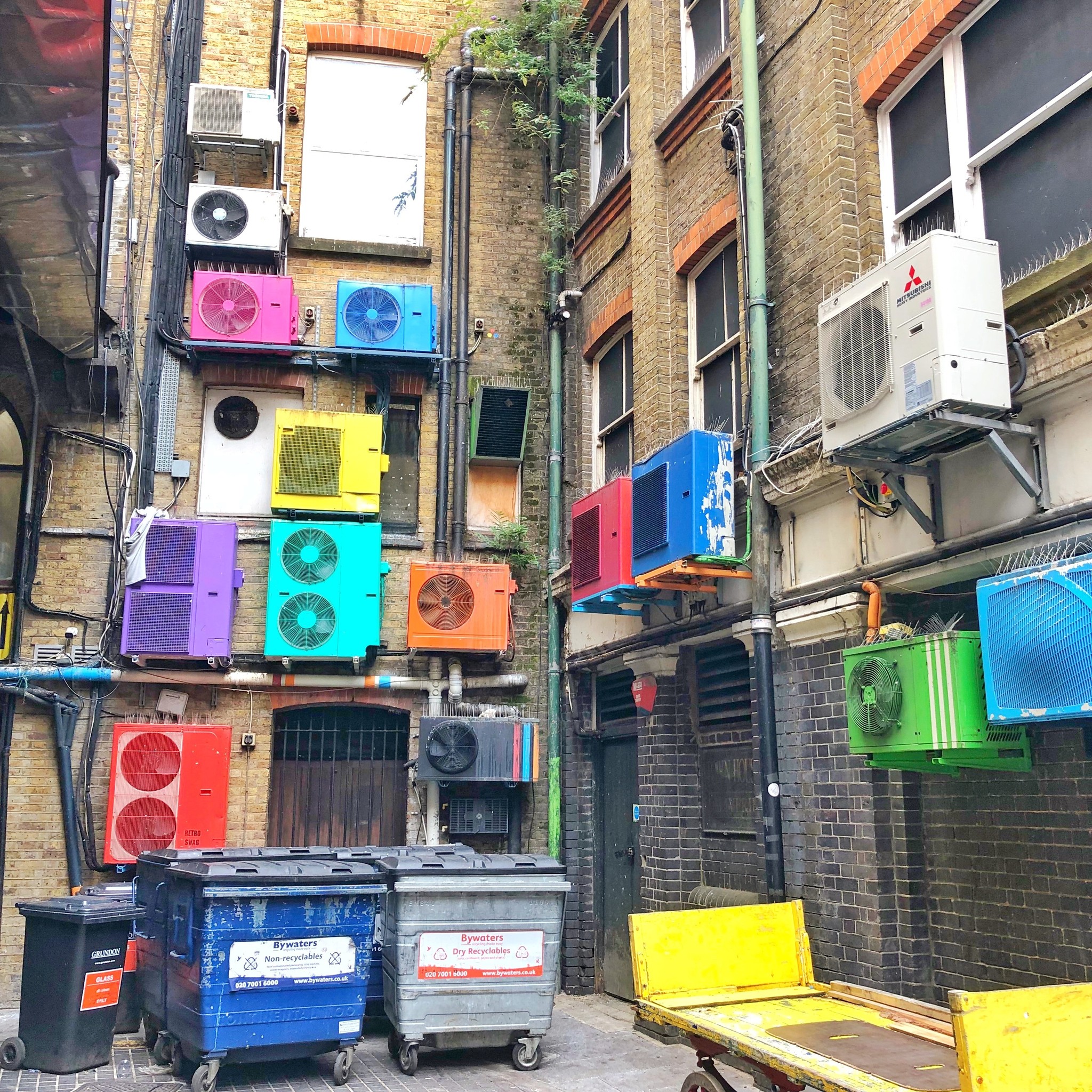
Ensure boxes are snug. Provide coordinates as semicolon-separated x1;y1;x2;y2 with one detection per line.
132;843;571;1092
0;898;144;1071
88;883;144;1032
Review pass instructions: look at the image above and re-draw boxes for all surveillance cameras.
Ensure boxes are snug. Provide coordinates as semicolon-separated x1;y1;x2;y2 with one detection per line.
65;627;78;639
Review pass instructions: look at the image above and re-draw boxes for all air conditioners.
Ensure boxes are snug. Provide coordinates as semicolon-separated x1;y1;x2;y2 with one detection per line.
103;723;231;863
842;629;1026;762
270;408;390;521
191;271;299;357
416;715;540;782
118;518;245;665
186;83;281;155
975;552;1092;726
185;183;283;264
406;560;516;651
336;279;438;352
262;519;393;667
630;430;736;577
569;477;634;603
816;229;1012;454
